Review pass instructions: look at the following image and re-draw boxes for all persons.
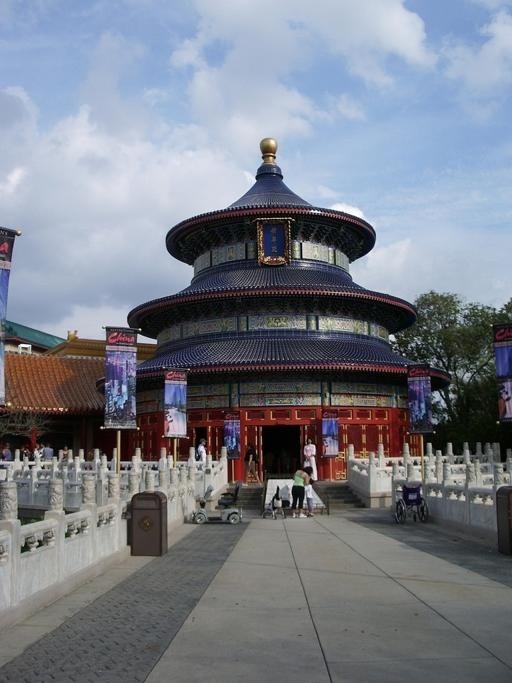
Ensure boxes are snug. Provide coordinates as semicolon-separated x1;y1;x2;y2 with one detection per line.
243;442;263;485
2;441;53;462
292;467;311;519
302;439;321;481
304;479;313;514
196;439;207;460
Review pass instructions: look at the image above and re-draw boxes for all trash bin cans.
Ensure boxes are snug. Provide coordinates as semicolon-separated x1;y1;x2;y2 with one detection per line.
130;489;169;558
495;485;512;556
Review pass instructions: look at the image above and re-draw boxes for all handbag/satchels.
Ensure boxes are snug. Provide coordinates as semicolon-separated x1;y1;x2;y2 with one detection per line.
303;461;309;468
255;455;258;463
195;449;201;459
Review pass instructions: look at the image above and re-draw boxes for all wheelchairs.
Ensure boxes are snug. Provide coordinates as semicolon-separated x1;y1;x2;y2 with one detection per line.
392;481;429;525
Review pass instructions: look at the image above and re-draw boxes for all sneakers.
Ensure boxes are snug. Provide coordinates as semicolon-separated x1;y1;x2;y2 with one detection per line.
308;512;314;516
299;513;307;517
293;513;298;518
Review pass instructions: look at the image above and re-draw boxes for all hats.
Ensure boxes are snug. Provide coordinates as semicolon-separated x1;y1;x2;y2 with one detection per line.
200;439;208;442
